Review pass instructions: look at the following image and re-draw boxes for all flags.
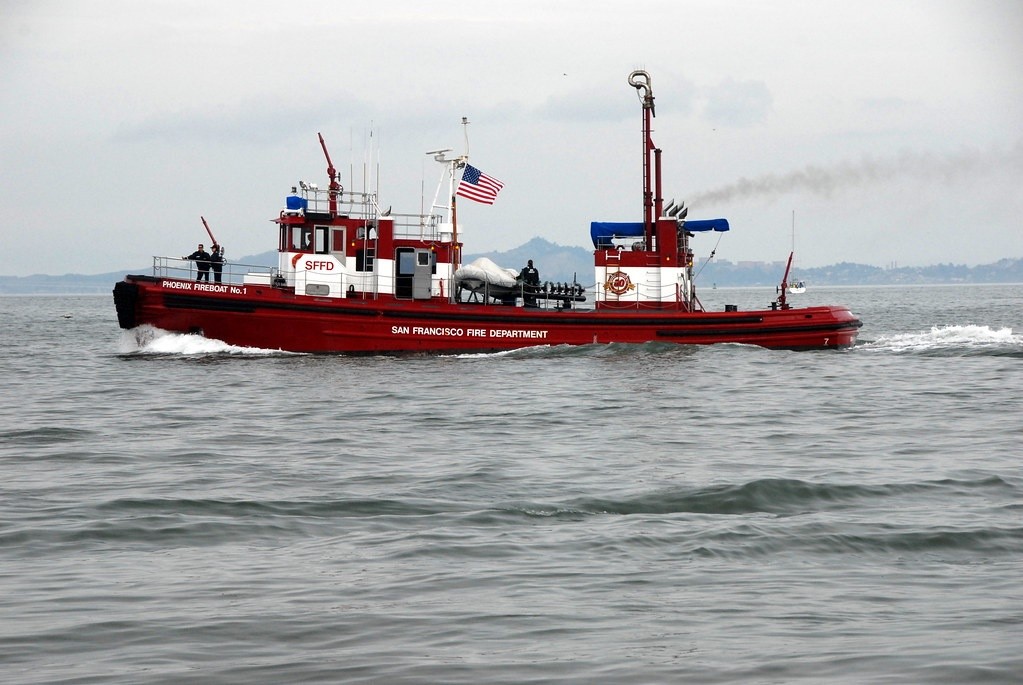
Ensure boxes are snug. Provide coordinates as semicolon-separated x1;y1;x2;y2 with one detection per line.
456;163;504;205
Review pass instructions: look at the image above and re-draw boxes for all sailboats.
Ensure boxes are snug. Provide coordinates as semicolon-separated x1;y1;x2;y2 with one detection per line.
788;209;807;293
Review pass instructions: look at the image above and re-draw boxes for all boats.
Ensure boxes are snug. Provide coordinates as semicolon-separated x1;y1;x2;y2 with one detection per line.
109;70;864;354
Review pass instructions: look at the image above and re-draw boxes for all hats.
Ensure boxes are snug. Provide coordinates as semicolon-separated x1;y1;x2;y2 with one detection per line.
211;245;216;248
198;244;203;248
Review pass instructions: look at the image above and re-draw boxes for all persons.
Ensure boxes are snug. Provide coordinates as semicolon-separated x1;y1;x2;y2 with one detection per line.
305;234;311;250
209;246;222;282
182;244;211;283
515;260;539;307
